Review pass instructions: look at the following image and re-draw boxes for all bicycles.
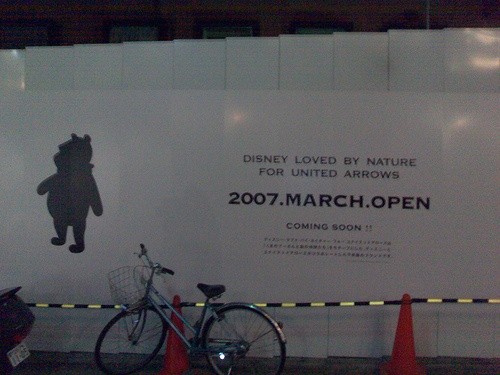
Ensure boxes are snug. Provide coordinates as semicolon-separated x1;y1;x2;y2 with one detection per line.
92;243;288;374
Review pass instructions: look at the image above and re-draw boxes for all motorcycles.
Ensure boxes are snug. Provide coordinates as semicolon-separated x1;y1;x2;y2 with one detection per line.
0;285;38;367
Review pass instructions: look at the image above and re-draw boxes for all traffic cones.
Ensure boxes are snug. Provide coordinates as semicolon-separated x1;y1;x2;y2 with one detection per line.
381;294;428;374
154;296;194;374
